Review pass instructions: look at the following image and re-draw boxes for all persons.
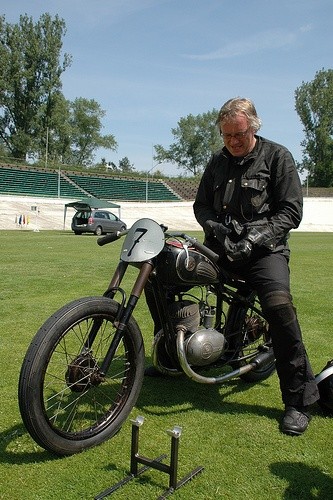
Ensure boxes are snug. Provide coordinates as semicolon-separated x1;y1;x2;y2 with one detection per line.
144;98;320;436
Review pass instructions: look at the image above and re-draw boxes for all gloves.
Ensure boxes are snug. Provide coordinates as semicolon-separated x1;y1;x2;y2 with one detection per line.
209;223;236;262
232;228;264;263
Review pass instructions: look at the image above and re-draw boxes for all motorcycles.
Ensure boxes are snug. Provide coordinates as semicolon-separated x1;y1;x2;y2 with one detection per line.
18;218;277;455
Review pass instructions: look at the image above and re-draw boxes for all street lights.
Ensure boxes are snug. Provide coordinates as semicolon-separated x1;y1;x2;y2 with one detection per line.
146;160;163;203
58;164;67;200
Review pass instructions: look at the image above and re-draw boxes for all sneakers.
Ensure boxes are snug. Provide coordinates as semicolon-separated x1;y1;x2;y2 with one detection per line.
144;363;175;383
280;405;315;435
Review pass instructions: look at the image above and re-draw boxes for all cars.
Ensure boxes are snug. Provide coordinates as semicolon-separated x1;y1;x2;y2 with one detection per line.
71;203;127;235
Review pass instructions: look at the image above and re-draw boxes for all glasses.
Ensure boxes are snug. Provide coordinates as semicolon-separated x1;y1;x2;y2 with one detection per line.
220;126;251;139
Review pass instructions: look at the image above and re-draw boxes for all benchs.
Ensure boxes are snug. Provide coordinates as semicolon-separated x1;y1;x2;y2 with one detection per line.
0;167;182;202
